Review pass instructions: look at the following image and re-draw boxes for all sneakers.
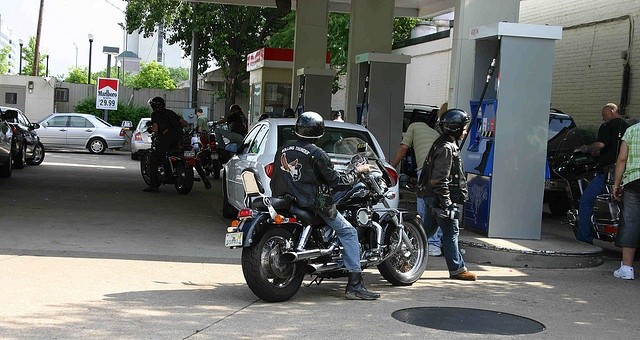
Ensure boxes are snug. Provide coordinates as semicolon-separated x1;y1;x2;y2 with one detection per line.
202;176;211;189
428;244;443;256
613;261;635;280
142;186;158;192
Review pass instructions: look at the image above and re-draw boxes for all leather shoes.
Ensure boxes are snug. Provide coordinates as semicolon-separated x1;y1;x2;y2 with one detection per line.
450;271;476;281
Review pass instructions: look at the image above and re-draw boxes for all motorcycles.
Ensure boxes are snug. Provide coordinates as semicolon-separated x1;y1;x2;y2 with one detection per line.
224;137;429;303
196;122;222;179
551;126;622;245
140;121;211;195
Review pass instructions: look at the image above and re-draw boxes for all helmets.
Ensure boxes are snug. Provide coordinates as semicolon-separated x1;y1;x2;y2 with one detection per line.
439;109;470;137
147;97;165;108
295;111;324;139
229;104;241;113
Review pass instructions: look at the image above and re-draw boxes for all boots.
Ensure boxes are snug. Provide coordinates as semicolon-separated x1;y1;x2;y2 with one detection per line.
345;272;380;299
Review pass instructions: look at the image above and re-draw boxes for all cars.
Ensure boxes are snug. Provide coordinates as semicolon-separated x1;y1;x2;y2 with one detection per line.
219;117;400;220
130;117;154;160
33;112;126;154
0;107;45;169
0;109;15;178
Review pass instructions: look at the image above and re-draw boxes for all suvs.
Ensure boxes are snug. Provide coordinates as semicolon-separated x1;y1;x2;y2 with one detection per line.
543;108;579;215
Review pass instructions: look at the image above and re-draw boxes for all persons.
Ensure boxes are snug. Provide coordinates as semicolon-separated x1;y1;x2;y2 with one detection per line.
333;111;344;123
418;109;477;280
270;111;380;300
142;96;189;192
390;121;443;256
212;104;248;150
195;107;211;139
579;103;629;245
612;121;640;279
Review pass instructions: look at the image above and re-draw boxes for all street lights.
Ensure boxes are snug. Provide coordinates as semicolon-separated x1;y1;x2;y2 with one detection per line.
18;39;23;75
88;34;93;83
117;61;121;78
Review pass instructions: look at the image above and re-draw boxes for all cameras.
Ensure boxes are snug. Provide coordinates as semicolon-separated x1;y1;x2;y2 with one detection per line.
430;205;460;219
